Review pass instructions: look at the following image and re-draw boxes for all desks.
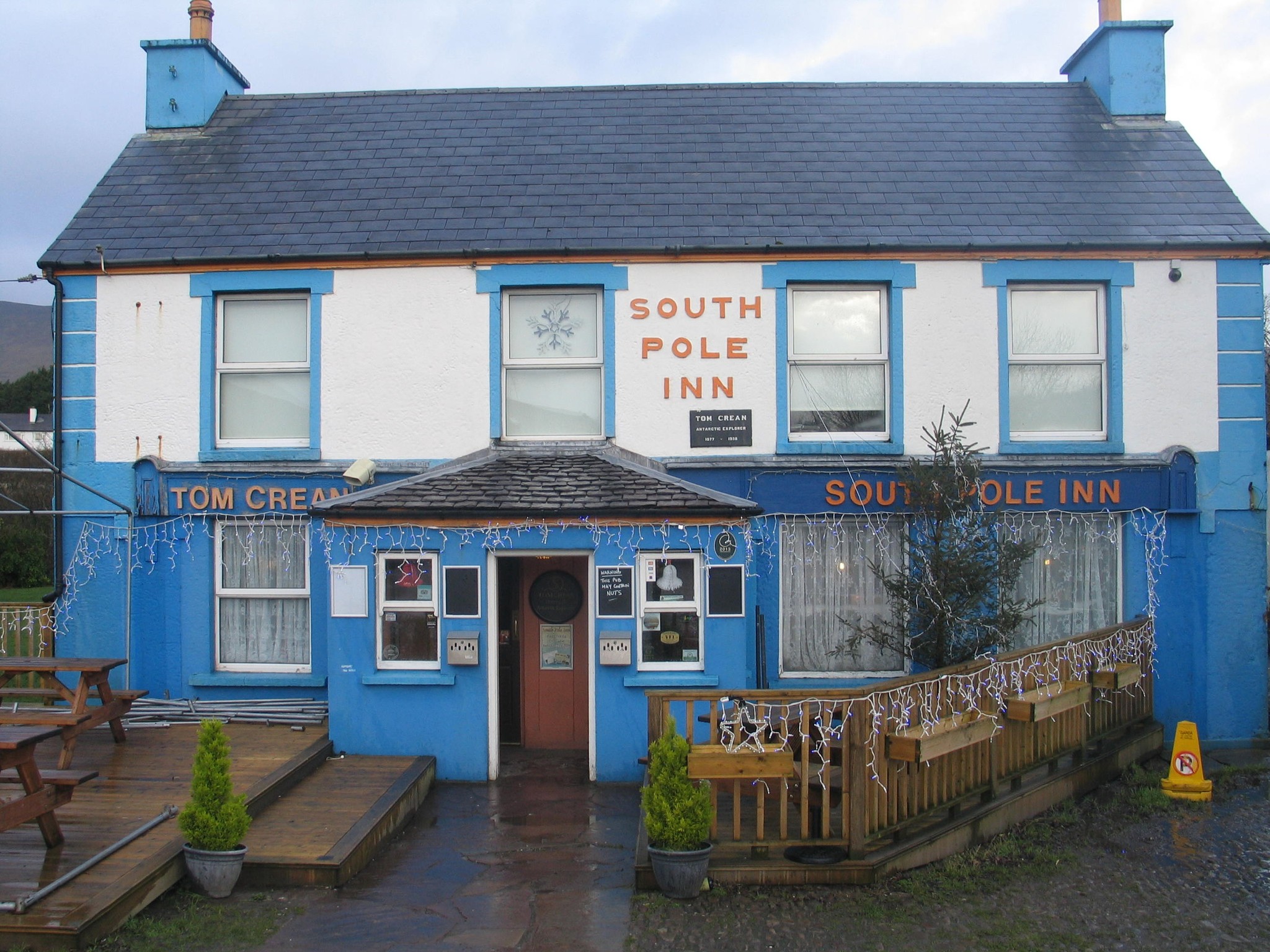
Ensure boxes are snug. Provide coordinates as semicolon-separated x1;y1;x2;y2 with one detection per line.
0;656;129;769
0;726;73;847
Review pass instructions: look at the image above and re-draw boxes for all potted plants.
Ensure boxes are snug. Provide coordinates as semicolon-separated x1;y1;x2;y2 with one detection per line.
640;713;713;899
180;719;254;899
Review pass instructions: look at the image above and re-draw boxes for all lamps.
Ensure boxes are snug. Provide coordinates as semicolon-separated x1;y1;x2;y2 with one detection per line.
343;459;376;485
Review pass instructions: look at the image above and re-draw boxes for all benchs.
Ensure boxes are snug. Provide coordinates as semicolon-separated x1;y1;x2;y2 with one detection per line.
0;711;92;724
0;768;99;785
0;686;150;700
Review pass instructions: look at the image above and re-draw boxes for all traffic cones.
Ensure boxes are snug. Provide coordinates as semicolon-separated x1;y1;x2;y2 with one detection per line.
1161;721;1212;803
